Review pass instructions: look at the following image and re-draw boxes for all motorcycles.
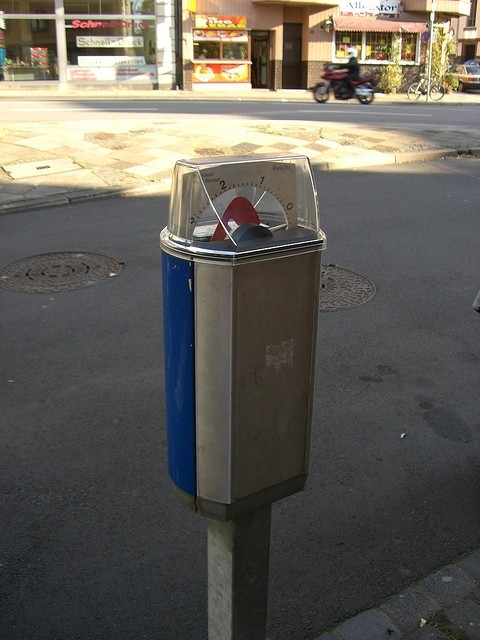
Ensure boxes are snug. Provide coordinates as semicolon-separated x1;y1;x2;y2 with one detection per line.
313;61;378;104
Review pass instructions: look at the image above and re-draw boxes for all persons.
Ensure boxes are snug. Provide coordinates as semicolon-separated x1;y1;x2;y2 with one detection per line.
333;48;359;97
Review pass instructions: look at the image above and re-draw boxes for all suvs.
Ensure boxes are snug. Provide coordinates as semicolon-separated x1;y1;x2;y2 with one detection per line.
464;60;480;64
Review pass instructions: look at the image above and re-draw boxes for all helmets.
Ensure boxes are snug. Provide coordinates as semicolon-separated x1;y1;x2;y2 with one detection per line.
348;47;358;59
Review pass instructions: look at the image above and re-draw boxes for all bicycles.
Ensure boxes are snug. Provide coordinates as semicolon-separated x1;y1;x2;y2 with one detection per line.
407;72;445;101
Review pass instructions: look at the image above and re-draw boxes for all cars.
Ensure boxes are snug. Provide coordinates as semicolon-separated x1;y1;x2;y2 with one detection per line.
446;64;480;92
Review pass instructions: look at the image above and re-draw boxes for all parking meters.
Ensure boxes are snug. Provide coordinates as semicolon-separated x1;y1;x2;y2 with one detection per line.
157;153;328;640
